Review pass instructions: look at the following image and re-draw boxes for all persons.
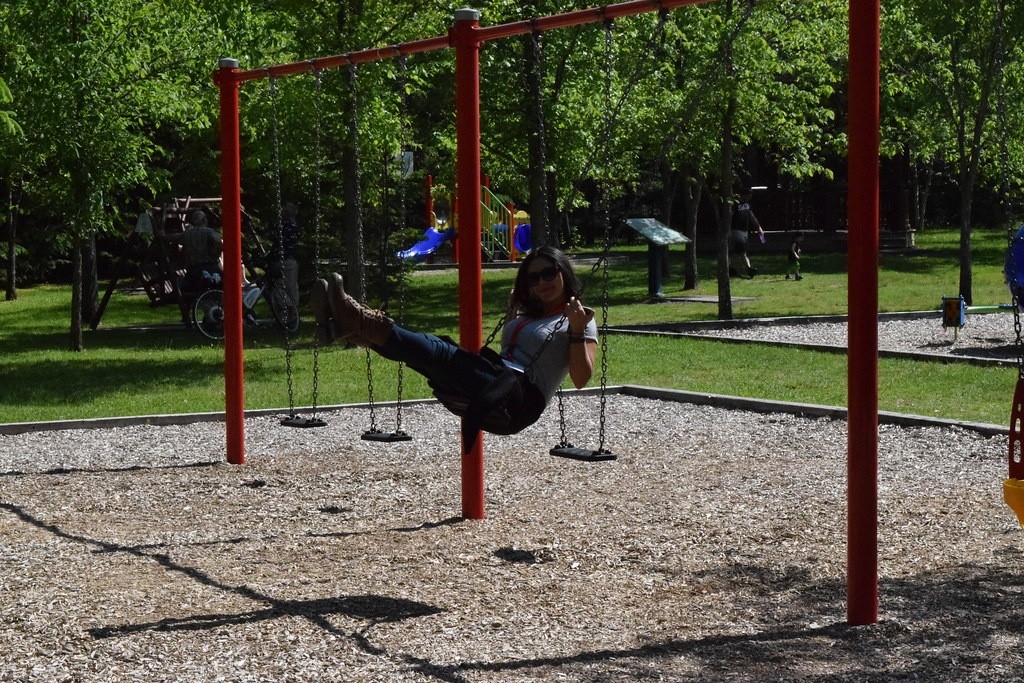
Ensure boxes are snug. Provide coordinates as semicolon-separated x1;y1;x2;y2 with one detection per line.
786;230;805;281
171;211;224;328
729;188;764;278
311;244;598;435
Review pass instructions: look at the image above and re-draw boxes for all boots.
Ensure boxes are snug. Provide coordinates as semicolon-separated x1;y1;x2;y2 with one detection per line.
311;271;394;347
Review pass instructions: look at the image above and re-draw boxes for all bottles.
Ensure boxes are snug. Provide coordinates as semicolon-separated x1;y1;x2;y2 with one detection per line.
759;232;766;243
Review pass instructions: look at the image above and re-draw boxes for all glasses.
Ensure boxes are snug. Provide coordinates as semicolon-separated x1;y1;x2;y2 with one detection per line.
525;267;563;286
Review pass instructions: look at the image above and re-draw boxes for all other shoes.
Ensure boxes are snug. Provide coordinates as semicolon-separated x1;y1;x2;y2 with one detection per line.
749;268;756;277
786;275;789;279
796;276;802;280
728;267;738;277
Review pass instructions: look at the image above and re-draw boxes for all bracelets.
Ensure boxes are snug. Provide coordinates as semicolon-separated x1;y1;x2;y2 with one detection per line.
571;331;587;336
570;337;585;342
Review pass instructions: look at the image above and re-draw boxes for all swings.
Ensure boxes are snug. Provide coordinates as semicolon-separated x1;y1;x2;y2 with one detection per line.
270;71;328;428
347;55;413;443
992;52;1024;528
462;0;757;435
530;19;618;463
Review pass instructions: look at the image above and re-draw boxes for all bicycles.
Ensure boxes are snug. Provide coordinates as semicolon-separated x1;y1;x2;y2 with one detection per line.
193;247;300;340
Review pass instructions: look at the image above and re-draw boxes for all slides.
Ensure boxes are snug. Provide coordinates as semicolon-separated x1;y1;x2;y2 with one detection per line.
396;227;454;264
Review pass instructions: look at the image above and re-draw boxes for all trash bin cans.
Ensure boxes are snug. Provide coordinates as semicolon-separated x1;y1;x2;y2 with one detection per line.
942;294;966;329
